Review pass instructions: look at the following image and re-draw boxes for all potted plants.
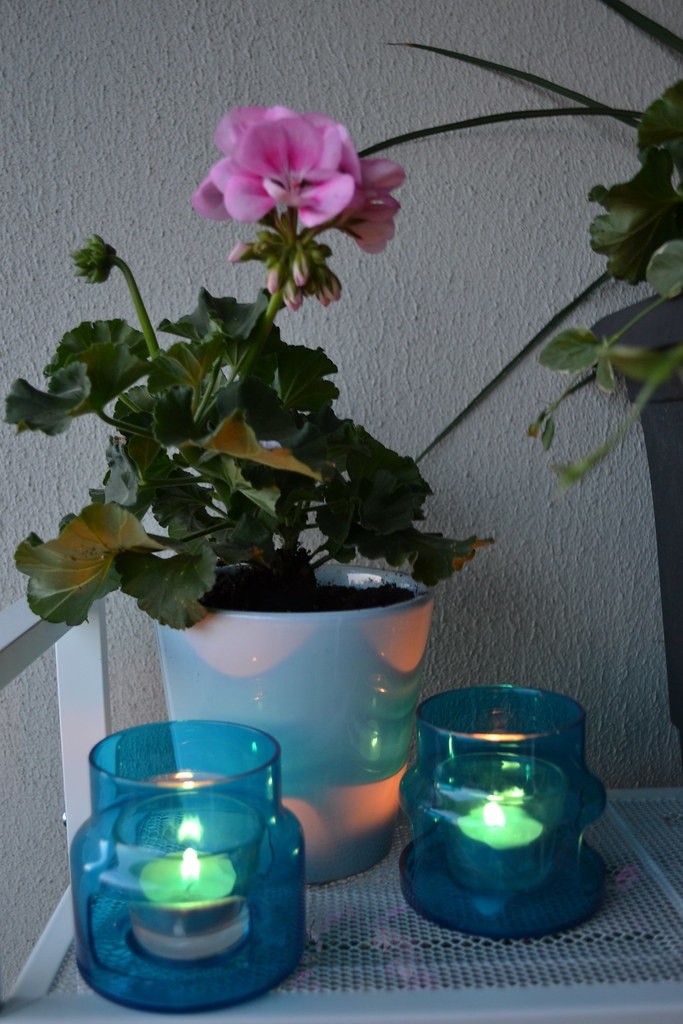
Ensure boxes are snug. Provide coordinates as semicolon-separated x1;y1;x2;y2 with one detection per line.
359;0;683;741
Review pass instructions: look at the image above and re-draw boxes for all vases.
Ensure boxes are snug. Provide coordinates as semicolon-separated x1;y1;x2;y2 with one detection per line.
156;563;436;884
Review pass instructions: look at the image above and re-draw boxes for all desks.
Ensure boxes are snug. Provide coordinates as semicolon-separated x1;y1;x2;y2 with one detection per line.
0;595;683;1024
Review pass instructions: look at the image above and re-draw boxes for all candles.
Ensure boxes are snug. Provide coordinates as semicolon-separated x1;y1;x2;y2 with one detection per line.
131;813;251;959
452;786;549;890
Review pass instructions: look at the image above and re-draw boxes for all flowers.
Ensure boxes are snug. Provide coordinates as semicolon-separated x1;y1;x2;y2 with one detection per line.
3;103;495;631
